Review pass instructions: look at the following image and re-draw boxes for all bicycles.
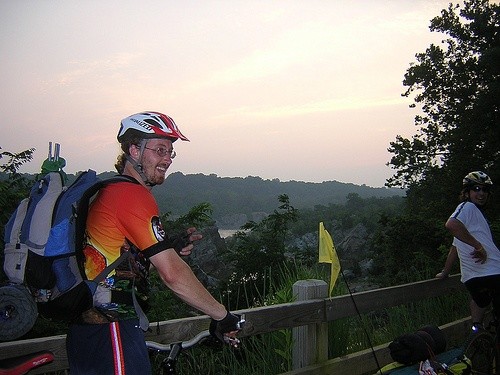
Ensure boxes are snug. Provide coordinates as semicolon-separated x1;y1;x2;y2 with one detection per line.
0;329;243;375
375;322;500;375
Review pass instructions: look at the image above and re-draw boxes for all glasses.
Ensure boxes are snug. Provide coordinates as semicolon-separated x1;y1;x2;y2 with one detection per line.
136;145;176;158
469;186;489;193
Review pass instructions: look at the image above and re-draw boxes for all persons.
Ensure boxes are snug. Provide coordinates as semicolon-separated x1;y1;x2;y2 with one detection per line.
65;112;241;375
436;171;500;375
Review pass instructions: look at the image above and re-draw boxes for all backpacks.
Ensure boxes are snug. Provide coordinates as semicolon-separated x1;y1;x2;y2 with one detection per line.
3;169;145;317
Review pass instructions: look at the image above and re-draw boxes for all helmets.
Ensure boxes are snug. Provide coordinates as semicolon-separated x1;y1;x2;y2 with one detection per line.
463;171;493;189
117;111;190;143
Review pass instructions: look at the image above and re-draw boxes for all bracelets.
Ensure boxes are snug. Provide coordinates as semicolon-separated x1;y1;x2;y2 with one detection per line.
475;245;482;251
